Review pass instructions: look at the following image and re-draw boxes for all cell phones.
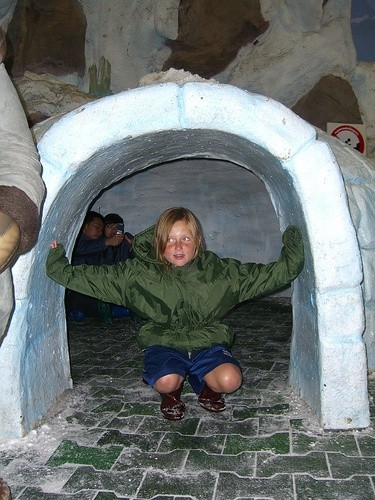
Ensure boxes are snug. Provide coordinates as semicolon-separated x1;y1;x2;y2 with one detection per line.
117;223;124;235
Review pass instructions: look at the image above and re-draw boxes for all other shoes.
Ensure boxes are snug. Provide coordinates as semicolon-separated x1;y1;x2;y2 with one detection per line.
157;383;185;419
198;381;226;412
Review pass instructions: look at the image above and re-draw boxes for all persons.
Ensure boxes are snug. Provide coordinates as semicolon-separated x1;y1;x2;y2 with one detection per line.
64;211;135;324
45;207;306;421
